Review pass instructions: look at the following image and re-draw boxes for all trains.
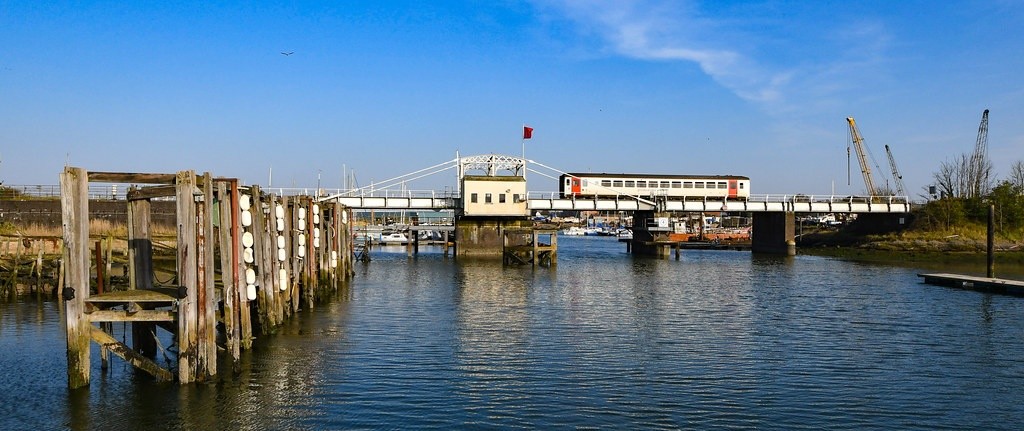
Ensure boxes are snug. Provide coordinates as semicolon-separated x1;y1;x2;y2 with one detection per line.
558;173;750;202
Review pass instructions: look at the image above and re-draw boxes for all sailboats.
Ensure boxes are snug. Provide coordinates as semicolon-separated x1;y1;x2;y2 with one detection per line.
563;211;634;243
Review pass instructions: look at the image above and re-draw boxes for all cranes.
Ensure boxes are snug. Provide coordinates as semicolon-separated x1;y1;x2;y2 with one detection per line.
846;115;906;201
967;108;990;198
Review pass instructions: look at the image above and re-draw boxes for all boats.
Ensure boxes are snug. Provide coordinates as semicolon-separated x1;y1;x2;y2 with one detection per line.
379;222;442;244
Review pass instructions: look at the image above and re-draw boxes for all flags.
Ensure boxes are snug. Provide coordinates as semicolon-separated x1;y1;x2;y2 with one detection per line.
524;127;533;139
